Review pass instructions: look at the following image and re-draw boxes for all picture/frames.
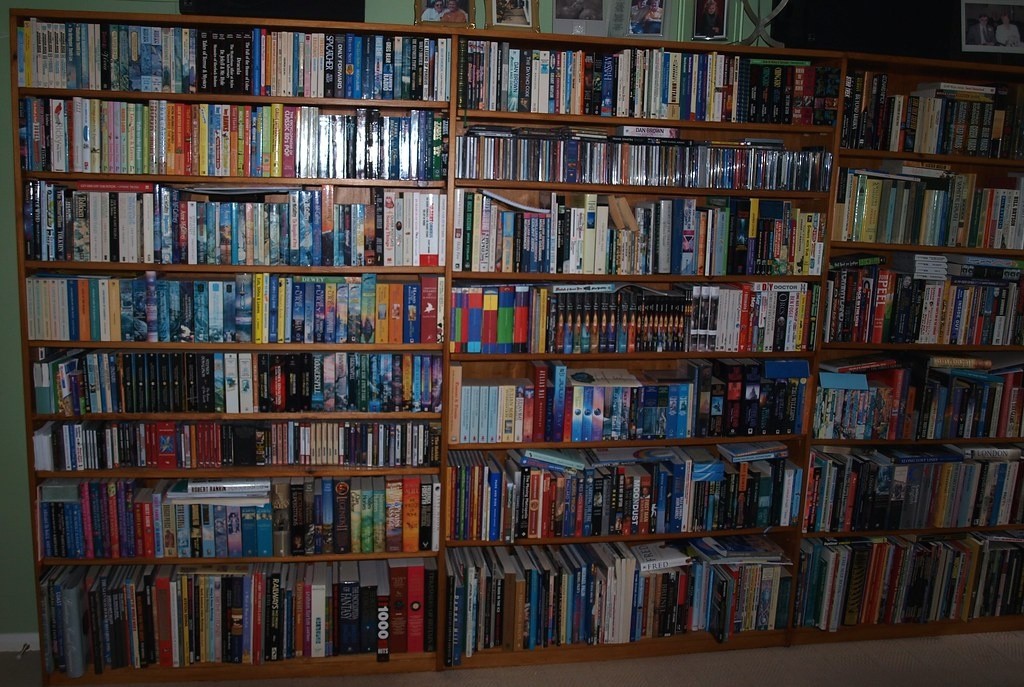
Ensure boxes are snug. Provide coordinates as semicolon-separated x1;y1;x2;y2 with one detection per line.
625;0;667;36
414;0;476;30
484;0;541;33
692;0;729;40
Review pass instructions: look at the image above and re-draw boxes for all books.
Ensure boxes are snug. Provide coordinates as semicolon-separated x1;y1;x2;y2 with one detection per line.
14;16;1024;679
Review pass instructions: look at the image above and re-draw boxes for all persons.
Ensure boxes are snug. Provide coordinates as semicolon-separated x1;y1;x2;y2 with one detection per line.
630;0;664;34
686;556;702;565
696;0;725;38
496;0;524;23
421;0;468;23
899;275;911;312
228;513;240;534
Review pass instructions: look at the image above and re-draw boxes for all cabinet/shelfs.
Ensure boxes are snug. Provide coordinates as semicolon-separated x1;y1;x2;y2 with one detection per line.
9;8;1024;687
